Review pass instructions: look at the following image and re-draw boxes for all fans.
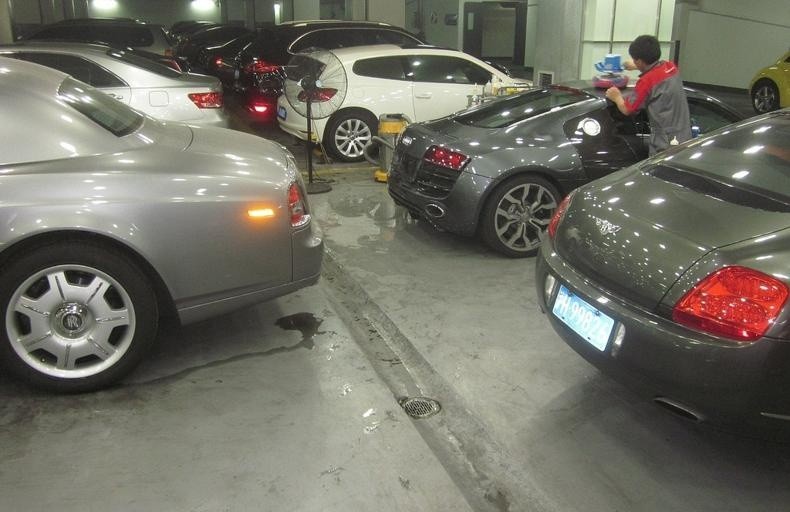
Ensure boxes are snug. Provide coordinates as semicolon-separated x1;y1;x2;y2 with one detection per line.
283;45;349;195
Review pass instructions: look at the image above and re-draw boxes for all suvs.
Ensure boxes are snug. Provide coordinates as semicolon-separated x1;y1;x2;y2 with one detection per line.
229;16;429;128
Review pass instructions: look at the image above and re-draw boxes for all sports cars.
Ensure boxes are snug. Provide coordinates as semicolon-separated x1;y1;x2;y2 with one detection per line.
391;81;751;261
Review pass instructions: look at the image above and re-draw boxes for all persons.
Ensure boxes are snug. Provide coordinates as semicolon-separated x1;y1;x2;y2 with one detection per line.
602;34;693;157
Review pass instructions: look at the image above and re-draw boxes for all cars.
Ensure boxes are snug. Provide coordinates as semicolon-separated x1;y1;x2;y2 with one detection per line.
0;16;261;89
1;55;326;394
278;41;537;163
749;50;790;118
536;107;788;444
1;40;230;129
